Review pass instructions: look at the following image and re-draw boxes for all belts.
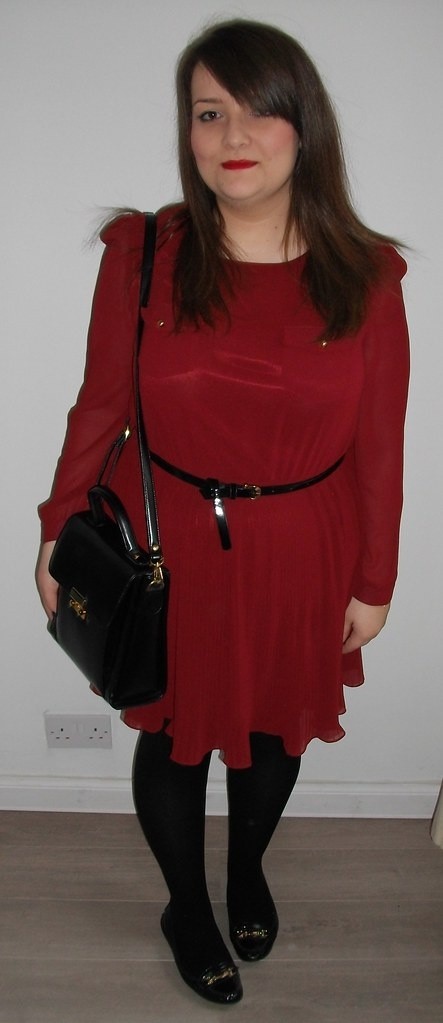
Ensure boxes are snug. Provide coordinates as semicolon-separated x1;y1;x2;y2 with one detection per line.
145;444;345;550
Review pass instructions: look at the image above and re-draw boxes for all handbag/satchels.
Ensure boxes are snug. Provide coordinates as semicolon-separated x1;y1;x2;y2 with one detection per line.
47;485;170;710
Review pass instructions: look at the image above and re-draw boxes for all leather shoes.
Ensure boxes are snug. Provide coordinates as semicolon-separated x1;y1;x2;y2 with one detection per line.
160;909;243;1005
226;875;279;962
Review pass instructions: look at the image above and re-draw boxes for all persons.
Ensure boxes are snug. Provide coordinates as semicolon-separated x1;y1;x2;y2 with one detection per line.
34;18;410;1005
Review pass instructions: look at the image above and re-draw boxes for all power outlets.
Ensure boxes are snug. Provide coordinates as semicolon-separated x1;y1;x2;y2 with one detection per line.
45;715;113;749
